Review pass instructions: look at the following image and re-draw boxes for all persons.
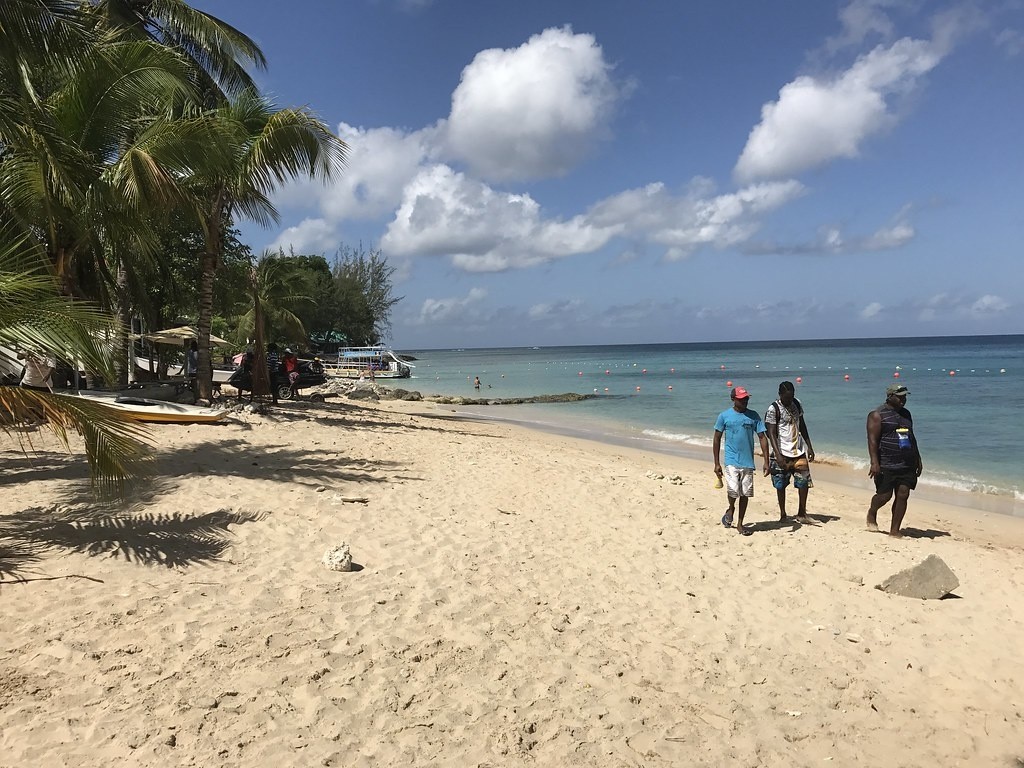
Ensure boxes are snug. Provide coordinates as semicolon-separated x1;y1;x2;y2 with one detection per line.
176;340;198;377
764;381;820;525
369;369;375;381
712;387;770;536
866;383;923;540
17;338;56;424
474;376;482;389
359;370;365;381
233;343;323;405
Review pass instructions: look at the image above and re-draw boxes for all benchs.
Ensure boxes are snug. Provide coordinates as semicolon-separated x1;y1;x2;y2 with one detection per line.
92;380;249;400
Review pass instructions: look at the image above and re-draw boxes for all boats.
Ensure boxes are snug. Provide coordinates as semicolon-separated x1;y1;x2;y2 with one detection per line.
321;346;416;377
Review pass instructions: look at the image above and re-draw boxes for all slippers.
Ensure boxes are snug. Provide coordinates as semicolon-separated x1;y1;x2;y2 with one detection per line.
736;525;752;536
722;506;735;528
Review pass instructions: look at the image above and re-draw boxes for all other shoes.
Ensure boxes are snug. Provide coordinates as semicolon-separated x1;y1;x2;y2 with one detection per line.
796;515;811;523
779;516;789;523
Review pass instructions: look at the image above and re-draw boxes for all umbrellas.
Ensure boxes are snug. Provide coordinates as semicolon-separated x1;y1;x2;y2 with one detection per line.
130;326;236;349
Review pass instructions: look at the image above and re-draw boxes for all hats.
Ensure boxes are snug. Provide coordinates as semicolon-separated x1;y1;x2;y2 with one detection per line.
885;383;912;400
730;386;752;399
283;348;293;353
